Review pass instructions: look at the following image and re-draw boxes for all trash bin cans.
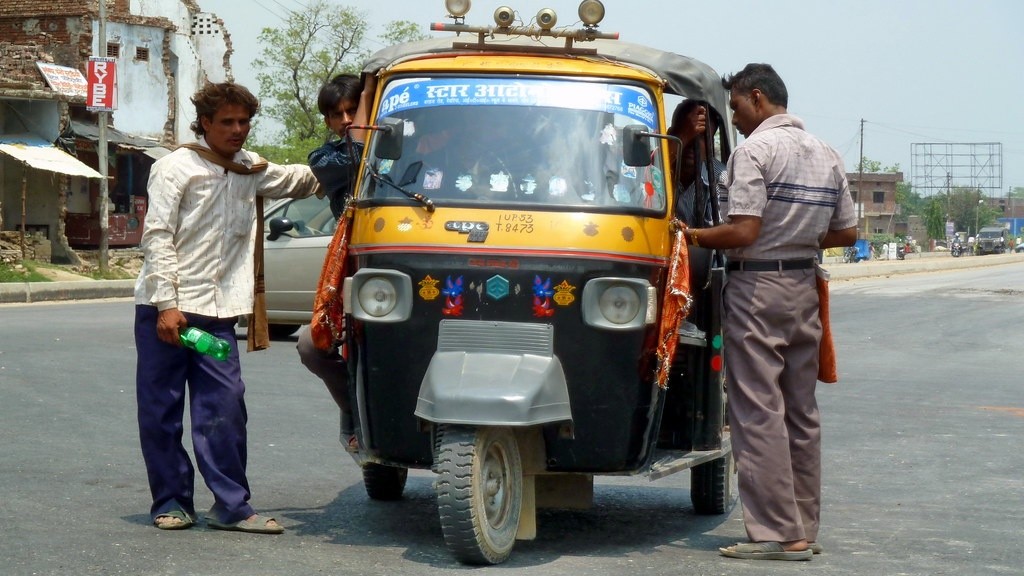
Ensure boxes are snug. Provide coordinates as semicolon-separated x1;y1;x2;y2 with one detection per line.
845;239;869;262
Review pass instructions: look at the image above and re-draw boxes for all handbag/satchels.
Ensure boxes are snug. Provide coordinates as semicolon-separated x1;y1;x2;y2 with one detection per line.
813;262;838;384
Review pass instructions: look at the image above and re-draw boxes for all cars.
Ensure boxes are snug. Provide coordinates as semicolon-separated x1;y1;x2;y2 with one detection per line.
254;191;340;338
1014;242;1024;253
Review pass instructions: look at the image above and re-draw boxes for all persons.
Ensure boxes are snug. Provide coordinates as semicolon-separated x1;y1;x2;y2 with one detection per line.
662;99;733;230
1015;236;1022;245
881;242;889;255
1007;239;1014;254
134;81;326;531
950;233;963;254
673;63;859;561
869;242;876;259
905;243;912;253
296;73;378;452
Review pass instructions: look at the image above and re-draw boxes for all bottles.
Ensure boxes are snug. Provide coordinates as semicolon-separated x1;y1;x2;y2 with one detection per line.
176;324;231;361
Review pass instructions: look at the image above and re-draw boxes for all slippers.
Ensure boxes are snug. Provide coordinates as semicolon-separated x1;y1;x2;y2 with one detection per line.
719;541;825;561
154;510;194;529
205;509;281;532
345;434;359;452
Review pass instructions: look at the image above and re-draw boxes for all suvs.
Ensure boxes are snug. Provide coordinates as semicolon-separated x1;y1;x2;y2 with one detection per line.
977;227;1008;255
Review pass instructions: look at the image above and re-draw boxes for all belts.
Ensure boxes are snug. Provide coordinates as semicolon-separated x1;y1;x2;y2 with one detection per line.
726;257;815;272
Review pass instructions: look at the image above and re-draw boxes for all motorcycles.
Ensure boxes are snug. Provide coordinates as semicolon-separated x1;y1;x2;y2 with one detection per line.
896;239;906;260
310;0;741;565
842;246;858;263
951;234;986;258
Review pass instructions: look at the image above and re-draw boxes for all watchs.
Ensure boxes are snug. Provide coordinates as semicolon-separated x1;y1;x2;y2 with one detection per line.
690;228;700;248
